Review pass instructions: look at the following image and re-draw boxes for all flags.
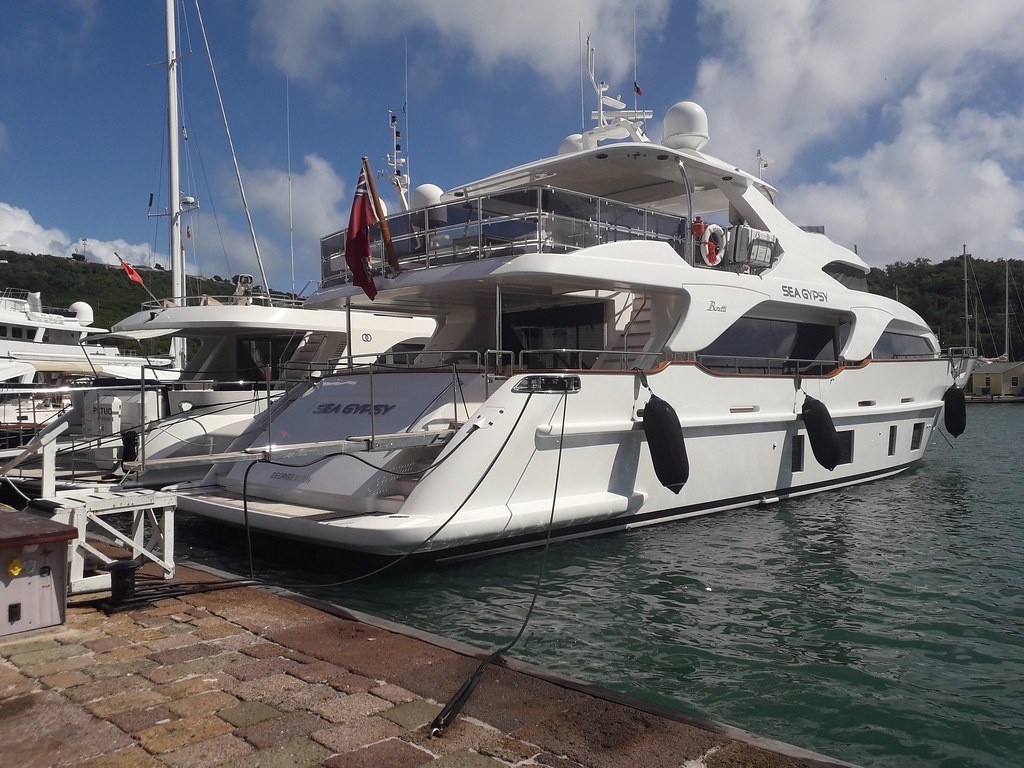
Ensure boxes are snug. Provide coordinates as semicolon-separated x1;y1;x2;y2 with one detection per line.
346;166;378;301
120;260;144;284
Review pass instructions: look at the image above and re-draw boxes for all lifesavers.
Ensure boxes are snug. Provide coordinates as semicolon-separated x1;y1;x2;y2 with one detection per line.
699;224;728;266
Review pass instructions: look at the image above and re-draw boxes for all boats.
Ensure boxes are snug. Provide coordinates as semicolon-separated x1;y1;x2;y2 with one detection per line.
149;32;955;566
0;300;439;490
0;295;175;437
